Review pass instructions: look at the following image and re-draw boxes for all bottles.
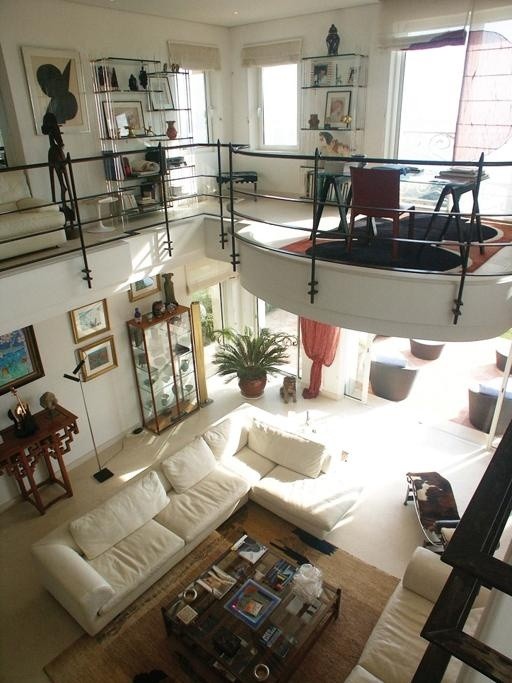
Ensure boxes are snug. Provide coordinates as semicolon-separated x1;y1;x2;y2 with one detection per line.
134;307;142;324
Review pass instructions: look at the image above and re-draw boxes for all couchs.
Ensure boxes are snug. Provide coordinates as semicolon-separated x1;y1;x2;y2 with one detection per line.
346;546;491;683
32;402;364;637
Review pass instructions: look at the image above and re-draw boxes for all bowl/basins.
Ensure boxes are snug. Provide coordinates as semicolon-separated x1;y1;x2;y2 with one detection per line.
143;328;198;421
166;155;186;167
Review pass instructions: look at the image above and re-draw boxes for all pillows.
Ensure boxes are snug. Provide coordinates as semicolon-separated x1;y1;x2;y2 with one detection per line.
68;416;332;559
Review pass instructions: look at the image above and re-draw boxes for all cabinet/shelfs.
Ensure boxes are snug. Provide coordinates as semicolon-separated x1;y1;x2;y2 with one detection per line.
325;90;352;129
300;53;368;131
125;306;200;434
147;70;195;171
90;56;167;141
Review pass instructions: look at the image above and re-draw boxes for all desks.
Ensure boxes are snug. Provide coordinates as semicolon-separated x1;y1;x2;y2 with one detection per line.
0;403;79;516
300;160;489;272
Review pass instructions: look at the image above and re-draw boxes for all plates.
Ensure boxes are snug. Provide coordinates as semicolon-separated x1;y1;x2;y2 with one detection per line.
450;167;478;174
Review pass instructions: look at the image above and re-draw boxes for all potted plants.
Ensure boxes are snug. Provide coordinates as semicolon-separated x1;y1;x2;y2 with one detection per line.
208;324;297;398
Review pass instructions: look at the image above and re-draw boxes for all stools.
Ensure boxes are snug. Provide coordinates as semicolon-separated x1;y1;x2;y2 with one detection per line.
85;197;119;233
371;336;511;434
217;172;257;203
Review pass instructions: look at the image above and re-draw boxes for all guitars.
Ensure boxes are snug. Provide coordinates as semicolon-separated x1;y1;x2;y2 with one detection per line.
7;388;38;435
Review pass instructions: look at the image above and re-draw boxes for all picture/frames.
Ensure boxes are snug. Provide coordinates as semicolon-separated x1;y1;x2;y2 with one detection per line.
148;76;174;110
102;101;146;139
20;45;90;135
0;273;162;396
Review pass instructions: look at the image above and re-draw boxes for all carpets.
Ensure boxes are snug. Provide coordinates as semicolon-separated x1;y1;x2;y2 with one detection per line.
43;499;400;683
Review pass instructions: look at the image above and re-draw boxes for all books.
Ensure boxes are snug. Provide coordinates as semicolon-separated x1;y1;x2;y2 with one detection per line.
430;167;489;187
306;161;359;205
104;154;167;216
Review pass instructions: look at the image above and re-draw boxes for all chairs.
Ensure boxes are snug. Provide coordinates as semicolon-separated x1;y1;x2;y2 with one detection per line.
405;470;461;553
0;170;67;262
348;166;415;257
